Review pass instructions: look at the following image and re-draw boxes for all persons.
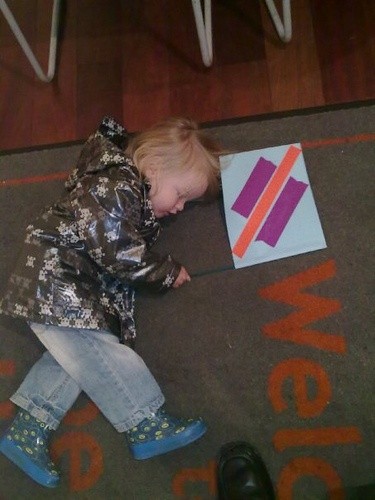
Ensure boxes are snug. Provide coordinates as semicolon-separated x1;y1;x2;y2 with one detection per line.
214;438;275;500
0;111;234;491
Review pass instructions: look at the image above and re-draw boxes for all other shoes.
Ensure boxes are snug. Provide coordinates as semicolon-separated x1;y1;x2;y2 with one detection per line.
0;406;62;488
125;408;208;462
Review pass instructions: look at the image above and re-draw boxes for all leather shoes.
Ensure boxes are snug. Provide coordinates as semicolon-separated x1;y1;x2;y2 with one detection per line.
217;442;275;500
